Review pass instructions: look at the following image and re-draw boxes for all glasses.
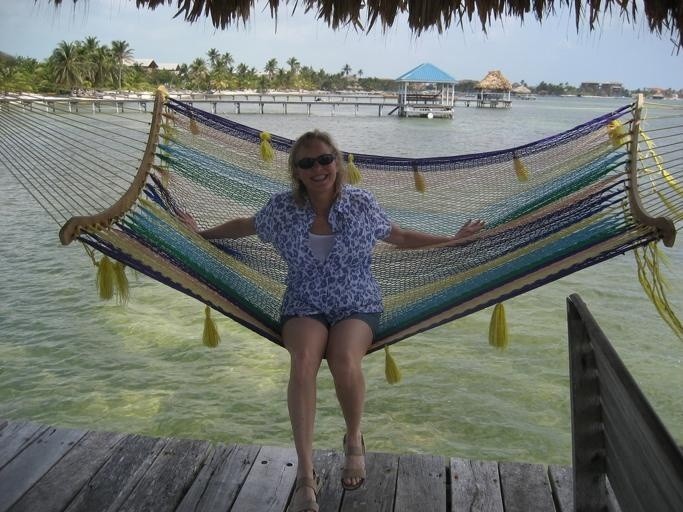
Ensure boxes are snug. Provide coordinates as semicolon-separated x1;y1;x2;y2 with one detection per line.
295;153;337;169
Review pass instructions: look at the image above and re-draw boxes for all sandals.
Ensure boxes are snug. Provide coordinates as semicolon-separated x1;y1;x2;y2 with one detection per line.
339;430;368;491
288;469;325;512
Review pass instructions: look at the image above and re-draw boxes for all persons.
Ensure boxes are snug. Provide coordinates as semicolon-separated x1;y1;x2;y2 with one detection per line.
171;128;489;511
